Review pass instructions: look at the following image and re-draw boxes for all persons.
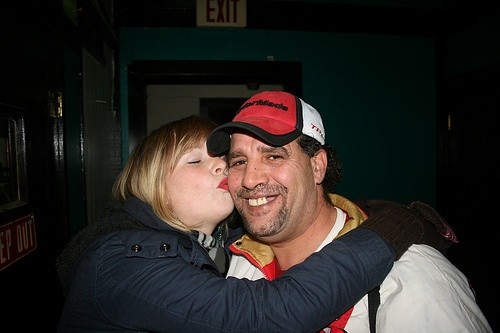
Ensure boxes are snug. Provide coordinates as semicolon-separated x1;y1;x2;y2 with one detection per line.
56;90;494;333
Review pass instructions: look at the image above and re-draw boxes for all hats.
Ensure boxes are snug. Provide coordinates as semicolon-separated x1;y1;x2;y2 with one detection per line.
207;90;325;155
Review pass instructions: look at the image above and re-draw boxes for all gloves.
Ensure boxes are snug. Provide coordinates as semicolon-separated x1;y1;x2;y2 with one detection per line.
322;142;344;193
358;200;458;261
